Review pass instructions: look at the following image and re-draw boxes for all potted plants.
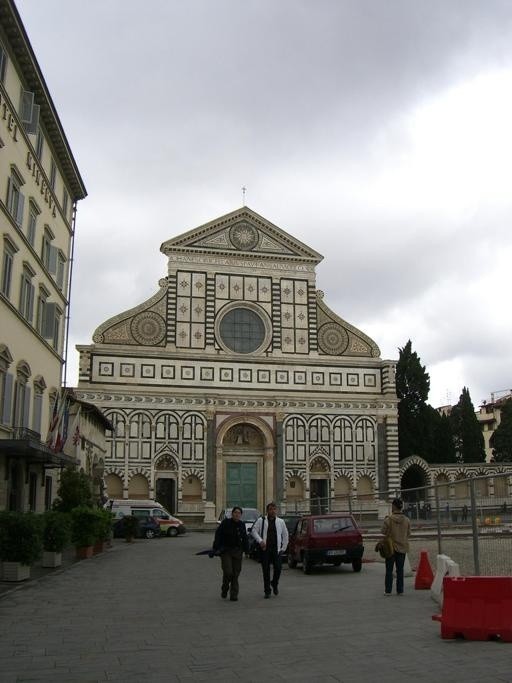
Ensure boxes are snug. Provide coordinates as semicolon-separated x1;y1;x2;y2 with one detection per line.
0;504;117;581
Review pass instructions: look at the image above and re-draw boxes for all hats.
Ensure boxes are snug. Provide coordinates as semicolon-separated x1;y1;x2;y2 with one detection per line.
393;498;403;509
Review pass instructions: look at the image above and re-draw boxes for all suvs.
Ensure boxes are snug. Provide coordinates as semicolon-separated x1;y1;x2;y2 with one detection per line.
215;507;364;573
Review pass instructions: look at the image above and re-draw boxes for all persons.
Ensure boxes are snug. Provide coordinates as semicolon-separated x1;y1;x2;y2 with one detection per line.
209;505;250;601
249;502;290;598
380;498;410;596
404;500;507;523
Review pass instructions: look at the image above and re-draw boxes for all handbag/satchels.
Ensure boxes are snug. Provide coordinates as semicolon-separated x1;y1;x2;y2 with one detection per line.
247;533;263;559
375;537;395;559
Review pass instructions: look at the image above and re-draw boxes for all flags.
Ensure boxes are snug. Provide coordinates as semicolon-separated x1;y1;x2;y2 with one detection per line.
45;389;83;458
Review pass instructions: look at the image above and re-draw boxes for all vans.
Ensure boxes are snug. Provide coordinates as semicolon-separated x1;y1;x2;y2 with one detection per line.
103;499;187;538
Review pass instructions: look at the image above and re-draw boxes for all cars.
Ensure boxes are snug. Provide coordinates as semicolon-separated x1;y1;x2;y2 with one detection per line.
112;515;161;539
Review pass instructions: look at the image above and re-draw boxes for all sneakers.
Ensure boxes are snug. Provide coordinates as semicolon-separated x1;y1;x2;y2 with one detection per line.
397;593;404;596
384;593;392;597
230;591;238;602
271;580;279;596
265;591;271;599
221;588;228;598
